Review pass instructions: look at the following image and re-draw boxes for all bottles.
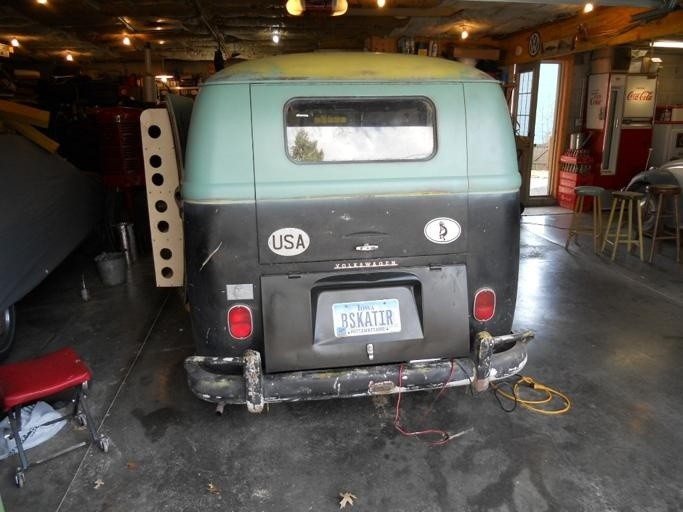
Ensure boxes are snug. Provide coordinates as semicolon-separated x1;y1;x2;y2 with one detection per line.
79;273;90;302
664;109;670;121
562;149;591;174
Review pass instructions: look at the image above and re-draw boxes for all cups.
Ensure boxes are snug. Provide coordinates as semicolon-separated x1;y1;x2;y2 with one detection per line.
109;222;136;255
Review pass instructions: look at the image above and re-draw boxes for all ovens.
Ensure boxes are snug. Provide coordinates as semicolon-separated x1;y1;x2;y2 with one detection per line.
651;124;683;167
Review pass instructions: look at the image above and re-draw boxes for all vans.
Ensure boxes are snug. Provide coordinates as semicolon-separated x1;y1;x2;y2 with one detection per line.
166;45;534;418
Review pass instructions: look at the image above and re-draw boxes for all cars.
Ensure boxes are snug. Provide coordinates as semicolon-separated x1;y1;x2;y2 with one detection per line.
621;158;682;238
0;126;128;361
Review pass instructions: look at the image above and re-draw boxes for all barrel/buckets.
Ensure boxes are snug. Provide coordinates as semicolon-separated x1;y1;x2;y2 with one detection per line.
111;221;141;265
570;132;593;150
96;104;144;192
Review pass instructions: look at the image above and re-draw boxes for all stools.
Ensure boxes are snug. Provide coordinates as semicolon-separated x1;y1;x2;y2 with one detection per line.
0;346;109;489
565;186;605;255
632;183;681;264
599;191;646;262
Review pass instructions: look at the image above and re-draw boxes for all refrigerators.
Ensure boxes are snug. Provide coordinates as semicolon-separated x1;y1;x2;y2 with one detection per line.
583;73;657;211
